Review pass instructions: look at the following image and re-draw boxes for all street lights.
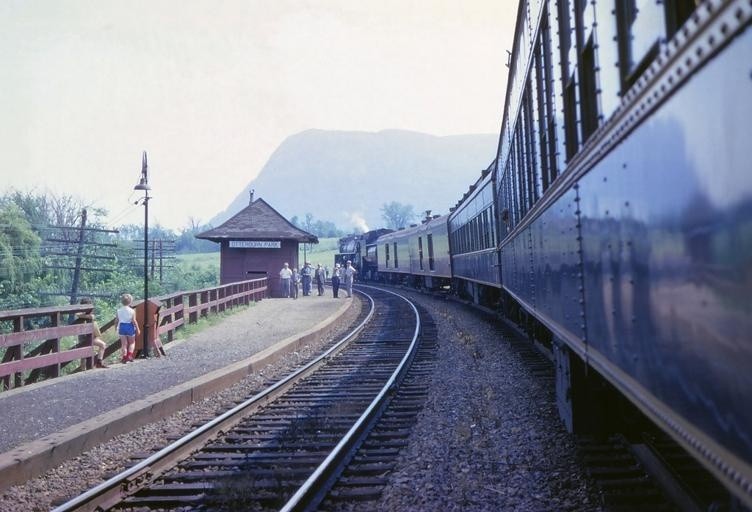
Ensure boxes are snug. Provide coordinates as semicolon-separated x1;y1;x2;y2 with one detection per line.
135;152;151;358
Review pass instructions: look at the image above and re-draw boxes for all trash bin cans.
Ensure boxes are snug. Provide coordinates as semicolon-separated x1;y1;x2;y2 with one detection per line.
132;299;167;359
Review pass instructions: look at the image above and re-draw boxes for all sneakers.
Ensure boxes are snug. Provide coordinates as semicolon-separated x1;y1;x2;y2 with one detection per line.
96;360;109;369
121;356;134;364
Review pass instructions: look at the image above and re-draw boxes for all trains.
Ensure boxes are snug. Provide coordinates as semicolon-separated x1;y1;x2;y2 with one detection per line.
335;1;751;511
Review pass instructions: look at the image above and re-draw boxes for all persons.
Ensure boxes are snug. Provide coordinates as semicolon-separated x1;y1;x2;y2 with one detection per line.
79;297;110;370
114;293;141;364
278;260;357;299
